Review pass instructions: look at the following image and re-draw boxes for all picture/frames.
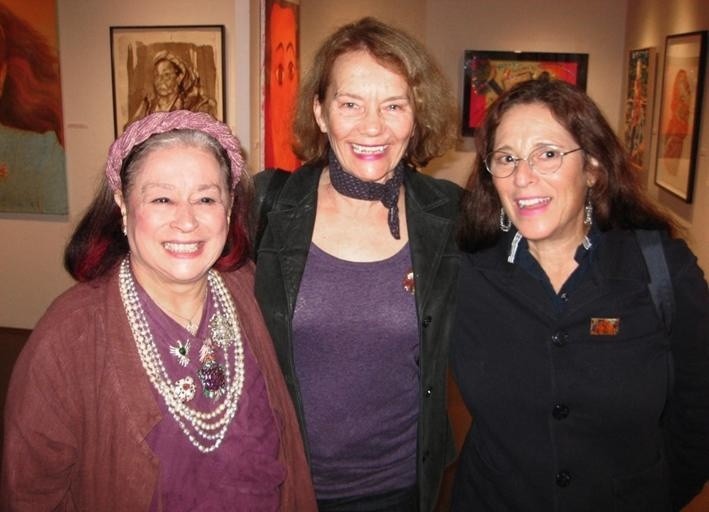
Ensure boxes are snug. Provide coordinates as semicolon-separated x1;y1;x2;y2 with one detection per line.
461;49;589;138
108;26;228;141
614;30;709;204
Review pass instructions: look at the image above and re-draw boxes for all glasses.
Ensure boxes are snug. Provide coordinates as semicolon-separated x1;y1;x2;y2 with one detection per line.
483;146;584;178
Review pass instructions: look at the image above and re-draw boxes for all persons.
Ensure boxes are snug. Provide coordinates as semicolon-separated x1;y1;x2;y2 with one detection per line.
237;17;465;511
447;70;709;512
0;109;321;511
122;48;217;134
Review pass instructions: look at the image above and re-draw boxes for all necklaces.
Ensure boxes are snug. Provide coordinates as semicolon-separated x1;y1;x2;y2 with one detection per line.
119;252;248;455
